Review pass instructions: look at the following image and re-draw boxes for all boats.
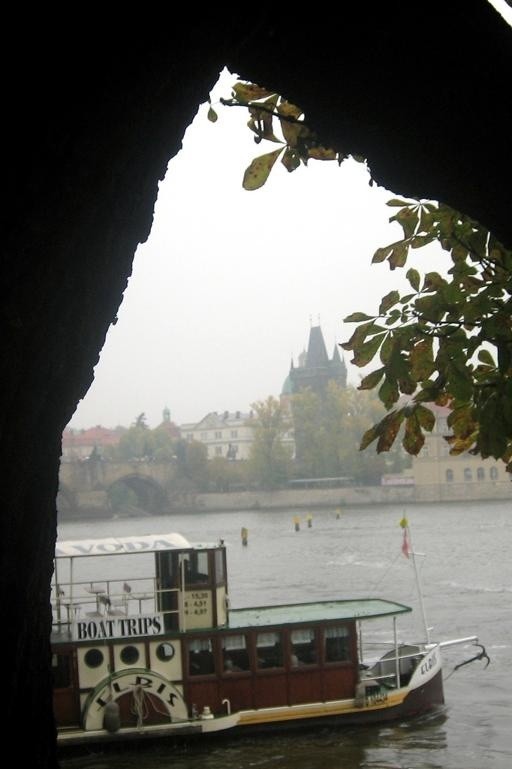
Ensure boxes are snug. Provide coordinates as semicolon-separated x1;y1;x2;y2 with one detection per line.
52;532;492;752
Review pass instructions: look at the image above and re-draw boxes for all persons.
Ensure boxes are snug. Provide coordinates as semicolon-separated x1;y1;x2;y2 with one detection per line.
180;559;197;584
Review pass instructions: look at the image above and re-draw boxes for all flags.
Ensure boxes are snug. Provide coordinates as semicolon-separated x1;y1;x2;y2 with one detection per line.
402;530;409;560
